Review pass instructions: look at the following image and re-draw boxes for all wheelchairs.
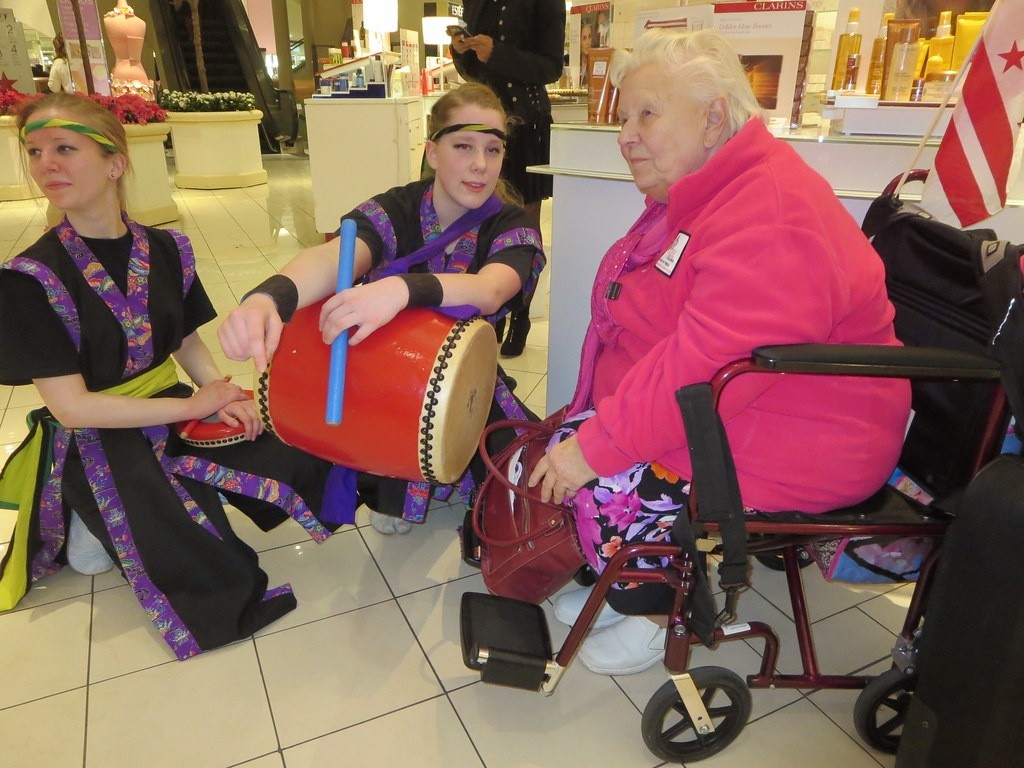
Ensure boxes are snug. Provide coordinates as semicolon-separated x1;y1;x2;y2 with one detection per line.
457;164;1024;763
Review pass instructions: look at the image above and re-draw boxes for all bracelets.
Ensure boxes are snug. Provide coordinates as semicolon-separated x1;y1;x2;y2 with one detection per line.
240;275;299;323
391;273;444;307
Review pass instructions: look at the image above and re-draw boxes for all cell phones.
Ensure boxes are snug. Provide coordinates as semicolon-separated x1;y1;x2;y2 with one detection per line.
447;25;473;42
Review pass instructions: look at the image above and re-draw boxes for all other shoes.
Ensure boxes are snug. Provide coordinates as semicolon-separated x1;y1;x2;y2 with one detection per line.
553;583;626;628
578;615;667;675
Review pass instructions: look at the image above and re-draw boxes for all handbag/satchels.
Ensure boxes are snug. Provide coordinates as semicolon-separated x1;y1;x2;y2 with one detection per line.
473;405;587;605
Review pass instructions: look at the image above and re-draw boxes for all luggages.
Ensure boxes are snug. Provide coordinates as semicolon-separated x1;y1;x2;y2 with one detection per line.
895;452;1024;768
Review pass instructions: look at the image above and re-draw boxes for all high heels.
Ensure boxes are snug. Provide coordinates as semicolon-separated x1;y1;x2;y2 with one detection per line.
494;316;506;343
500;316;531;357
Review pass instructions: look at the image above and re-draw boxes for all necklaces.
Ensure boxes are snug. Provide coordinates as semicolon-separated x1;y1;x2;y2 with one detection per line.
895;0;995;40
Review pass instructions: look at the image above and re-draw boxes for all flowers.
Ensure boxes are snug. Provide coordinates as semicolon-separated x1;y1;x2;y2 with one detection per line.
76;91;173;126
0;90;49;116
159;88;257;113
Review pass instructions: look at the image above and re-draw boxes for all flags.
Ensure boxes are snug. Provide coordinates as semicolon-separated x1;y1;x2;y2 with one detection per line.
921;0;1024;229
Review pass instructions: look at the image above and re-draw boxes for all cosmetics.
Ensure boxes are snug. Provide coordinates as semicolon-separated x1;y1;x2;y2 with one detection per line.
827;9;991;100
588;48;635;125
321;43;434;97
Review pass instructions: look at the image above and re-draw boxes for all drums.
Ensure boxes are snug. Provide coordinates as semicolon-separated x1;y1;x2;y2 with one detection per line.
173;389;261;449
253;291;499;493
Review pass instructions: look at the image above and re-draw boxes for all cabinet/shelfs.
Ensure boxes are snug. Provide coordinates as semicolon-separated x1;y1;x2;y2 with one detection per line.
304;97;425;242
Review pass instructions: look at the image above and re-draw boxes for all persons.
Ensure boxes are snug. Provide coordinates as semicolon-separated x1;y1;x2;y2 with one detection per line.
217;83;547;567
104;0;152;100
0;92;364;661
580;11;610;87
450;0;566;247
31;34;73;94
528;29;911;676
183;0;203;42
560;54;569;89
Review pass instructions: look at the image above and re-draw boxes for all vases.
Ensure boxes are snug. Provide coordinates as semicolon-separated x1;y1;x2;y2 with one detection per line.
166;109;268;189
0;116;46;200
46;118;178;229
